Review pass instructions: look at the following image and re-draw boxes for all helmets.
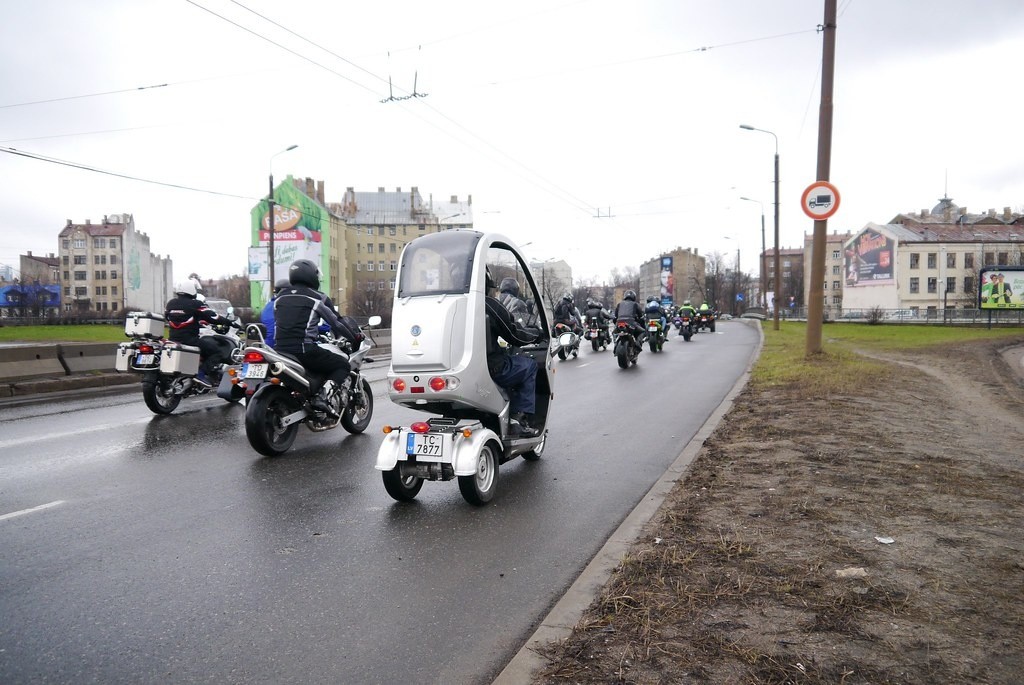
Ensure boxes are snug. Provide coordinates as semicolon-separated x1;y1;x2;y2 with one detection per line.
654;296;660;304
624;290;637;301
450;259;497;289
647;296;654;303
563;292;574;302
703;300;708;304
196;293;206;303
273;279;292;293
684;300;690;306
500;278;520;297
290;259;320;290
176;278;203;299
585;296;604;310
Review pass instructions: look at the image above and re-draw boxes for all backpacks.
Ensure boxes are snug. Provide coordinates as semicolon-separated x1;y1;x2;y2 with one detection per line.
645;300;660;313
554;300;569;319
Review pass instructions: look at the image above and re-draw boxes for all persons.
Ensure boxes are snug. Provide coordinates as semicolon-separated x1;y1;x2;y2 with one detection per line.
450;256;544;436
981;273;1012;304
165;279;240;388
261;258;365;419
661;268;673;298
552;290;716;350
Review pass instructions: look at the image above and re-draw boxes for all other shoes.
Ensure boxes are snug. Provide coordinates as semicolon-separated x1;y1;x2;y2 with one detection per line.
608;337;612;344
192;374;213;388
635;343;643;351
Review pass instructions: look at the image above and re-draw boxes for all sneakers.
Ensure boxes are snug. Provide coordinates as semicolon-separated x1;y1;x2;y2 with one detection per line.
507;422;541;438
311;386;340;420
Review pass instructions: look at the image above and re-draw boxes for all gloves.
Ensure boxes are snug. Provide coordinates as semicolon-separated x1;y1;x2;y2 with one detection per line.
226;319;241;329
526;325;545;344
352;332;365;348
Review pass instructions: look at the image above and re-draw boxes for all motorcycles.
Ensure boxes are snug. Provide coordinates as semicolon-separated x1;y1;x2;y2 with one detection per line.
551;323;581;360
583;309;612;351
374;227;556;506
114;307;250;415
229;315;382;456
611;314;670;369
673;315;715;341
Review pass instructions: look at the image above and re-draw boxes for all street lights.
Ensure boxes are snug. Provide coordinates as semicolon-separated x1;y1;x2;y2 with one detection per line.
740;124;780;330
741;197;768;317
269;144;299;302
